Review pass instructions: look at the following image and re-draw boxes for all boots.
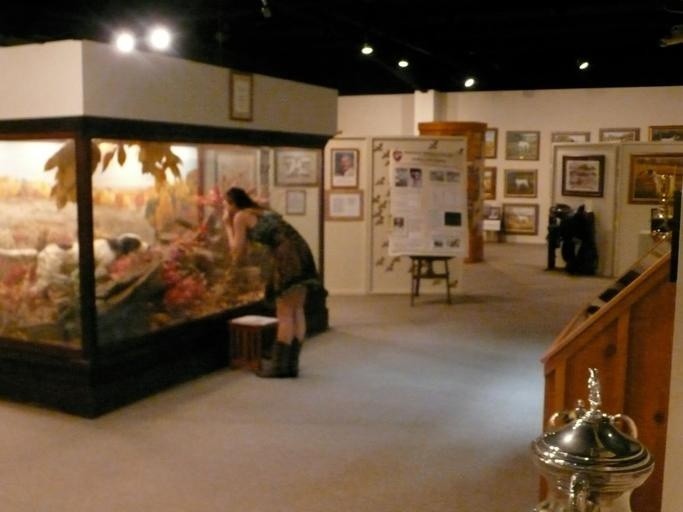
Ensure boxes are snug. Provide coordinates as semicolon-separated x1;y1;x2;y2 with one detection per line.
255;337;301;378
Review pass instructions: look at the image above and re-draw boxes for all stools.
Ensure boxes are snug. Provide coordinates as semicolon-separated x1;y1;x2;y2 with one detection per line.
227;314;279;372
407;250;454;308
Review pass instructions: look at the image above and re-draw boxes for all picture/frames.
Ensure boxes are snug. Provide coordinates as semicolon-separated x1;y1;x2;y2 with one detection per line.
272;145;323;214
229;69;254;123
561;154;605;199
482;127;540;244
323;147;365;222
629;153;683;205
548;125;683;146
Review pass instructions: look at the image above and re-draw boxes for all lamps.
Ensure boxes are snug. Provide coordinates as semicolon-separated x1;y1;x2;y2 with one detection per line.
569;46;594;72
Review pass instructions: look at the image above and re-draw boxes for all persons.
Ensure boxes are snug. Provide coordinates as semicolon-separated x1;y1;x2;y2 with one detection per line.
332;152;355;187
221;187;319;380
